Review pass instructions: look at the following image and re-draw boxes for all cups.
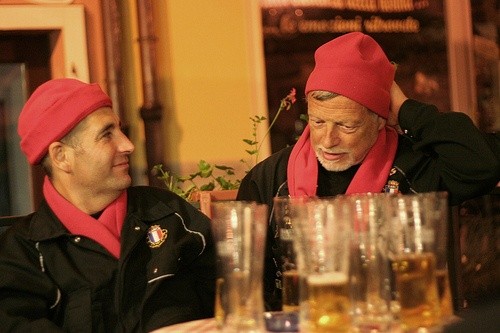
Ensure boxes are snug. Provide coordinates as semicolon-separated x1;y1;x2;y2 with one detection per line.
207;190;454;332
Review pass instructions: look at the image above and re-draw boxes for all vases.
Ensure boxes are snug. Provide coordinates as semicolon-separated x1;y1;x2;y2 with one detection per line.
210;190;238;202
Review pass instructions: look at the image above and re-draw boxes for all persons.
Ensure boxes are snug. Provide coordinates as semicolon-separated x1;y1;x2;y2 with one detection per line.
234;32;500;312
0;79;222;333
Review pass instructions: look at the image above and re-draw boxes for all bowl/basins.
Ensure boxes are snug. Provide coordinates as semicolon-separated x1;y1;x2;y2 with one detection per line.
263;311;299;332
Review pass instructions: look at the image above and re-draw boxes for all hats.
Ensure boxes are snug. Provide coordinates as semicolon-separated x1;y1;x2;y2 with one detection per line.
306;33;395;119
16;77;112;166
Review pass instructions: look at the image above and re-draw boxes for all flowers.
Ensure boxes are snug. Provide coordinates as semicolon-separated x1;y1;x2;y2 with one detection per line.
195;88;297;191
